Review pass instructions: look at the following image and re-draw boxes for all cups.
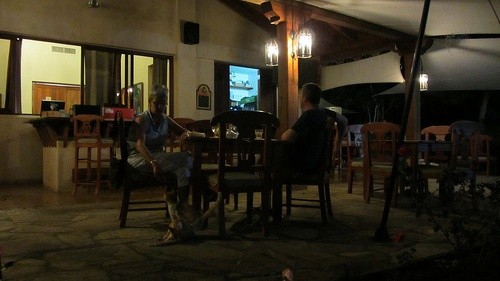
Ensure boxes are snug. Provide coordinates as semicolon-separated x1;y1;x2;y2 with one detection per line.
255;129;263;139
211;122;238;138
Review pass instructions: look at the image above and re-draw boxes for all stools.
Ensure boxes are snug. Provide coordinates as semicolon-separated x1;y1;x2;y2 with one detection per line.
72;114;113;202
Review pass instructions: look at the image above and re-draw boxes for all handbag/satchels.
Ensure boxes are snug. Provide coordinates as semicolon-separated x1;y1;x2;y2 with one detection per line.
158;152;188;172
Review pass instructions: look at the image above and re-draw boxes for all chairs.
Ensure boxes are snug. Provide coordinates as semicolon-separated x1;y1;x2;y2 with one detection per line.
111;105;494;242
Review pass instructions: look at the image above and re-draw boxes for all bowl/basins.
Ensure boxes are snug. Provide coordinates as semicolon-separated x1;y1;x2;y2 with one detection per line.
189;132;205;137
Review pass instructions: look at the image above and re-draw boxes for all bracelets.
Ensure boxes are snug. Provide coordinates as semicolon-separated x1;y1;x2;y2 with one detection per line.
150;159;157;163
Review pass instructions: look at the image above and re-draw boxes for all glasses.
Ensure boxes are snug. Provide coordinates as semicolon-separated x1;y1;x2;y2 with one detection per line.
151;101;168;105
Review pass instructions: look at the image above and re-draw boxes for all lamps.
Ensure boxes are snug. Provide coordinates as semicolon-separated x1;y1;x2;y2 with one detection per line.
418;57;430;92
264;21;280;67
294;12;315;58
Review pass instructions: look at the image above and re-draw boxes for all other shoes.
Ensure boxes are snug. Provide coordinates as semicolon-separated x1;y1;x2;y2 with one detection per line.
253;207;272;216
205;189;219;202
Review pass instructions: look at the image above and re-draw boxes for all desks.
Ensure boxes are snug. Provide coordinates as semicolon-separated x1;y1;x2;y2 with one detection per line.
183;131;282;228
366;137;452;205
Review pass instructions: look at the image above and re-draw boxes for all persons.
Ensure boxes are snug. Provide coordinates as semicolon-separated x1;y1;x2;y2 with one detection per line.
269;83;348;179
127;84;194;233
